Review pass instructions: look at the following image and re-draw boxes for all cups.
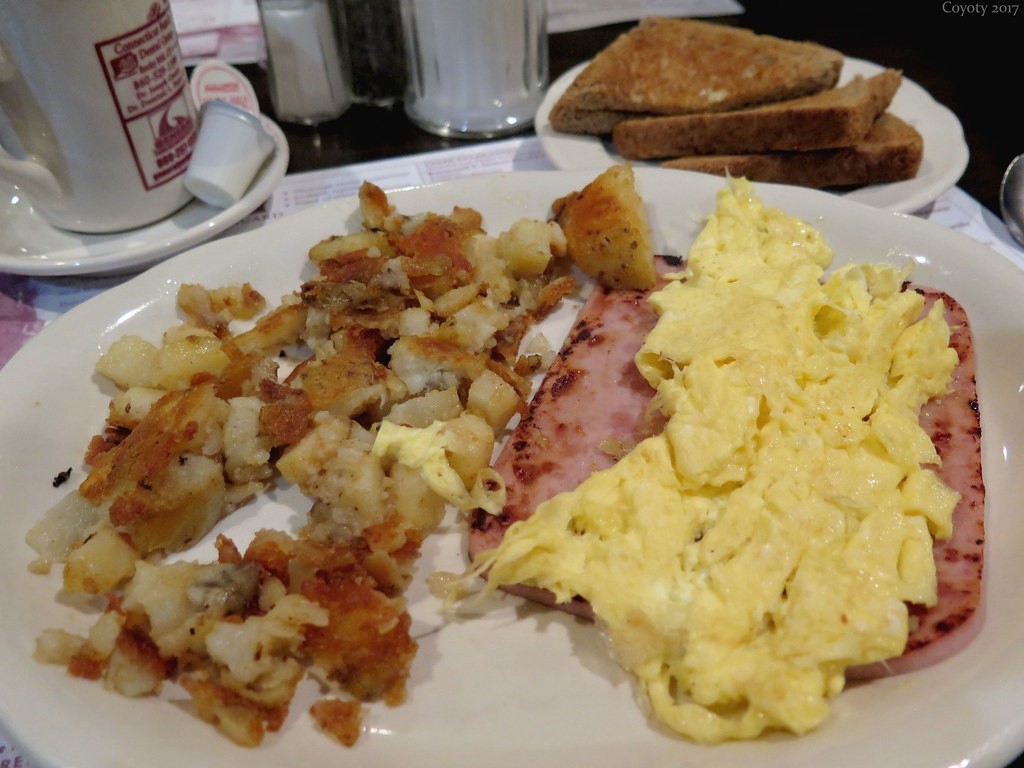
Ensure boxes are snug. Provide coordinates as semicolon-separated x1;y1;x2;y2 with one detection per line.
0;0;201;234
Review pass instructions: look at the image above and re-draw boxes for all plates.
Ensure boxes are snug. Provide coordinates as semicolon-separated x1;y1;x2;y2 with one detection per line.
534;55;970;215
0;113;290;276
0;169;1024;768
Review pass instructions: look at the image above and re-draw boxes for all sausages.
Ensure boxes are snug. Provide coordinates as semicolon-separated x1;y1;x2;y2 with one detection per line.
468;257;986;679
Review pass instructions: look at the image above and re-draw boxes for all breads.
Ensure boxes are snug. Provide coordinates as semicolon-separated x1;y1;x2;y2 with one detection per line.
546;17;923;191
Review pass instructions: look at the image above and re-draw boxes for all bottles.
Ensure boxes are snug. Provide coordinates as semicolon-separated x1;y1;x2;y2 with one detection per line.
346;0;406;108
399;0;549;138
258;0;353;125
